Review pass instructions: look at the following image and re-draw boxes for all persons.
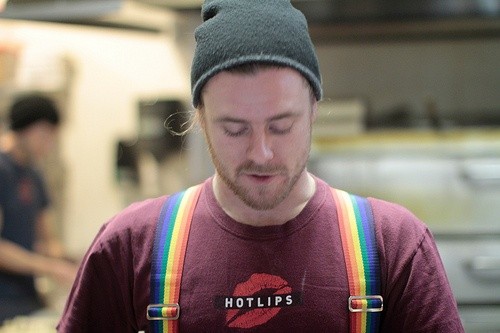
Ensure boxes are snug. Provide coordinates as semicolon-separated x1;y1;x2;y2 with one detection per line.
0;90;74;328
56;0;467;333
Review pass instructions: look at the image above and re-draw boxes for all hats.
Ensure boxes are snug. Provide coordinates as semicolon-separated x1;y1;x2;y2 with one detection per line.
190;0;322;109
